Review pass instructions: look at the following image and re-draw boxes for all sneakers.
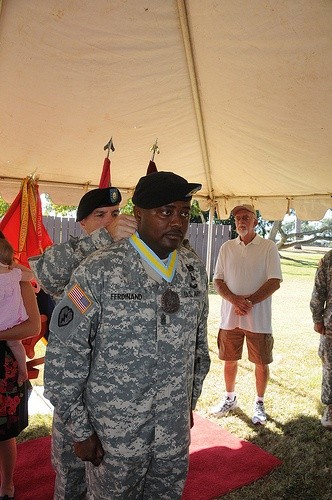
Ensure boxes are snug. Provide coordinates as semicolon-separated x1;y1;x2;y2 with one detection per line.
252;400;267;424
211;396;236;415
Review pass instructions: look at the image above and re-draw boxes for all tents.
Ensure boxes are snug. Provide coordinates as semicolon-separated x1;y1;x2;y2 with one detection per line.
0;0;332;286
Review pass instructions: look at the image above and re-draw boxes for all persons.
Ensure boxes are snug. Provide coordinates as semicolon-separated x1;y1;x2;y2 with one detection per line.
43;172;211;500
213;204;283;424
311;247;332;428
0;240;34;384
0;231;41;500
28;186;137;500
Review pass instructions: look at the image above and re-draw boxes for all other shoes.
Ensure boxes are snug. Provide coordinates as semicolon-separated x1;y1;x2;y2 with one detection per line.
322;405;332;428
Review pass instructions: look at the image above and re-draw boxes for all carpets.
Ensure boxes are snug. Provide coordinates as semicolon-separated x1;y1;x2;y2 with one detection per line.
13;411;286;500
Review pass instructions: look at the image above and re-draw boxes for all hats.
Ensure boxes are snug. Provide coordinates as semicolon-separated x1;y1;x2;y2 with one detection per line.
131;171;202;210
231;204;256;216
75;186;123;223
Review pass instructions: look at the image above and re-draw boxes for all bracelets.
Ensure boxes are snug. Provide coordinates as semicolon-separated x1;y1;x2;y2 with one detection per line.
246;299;252;304
